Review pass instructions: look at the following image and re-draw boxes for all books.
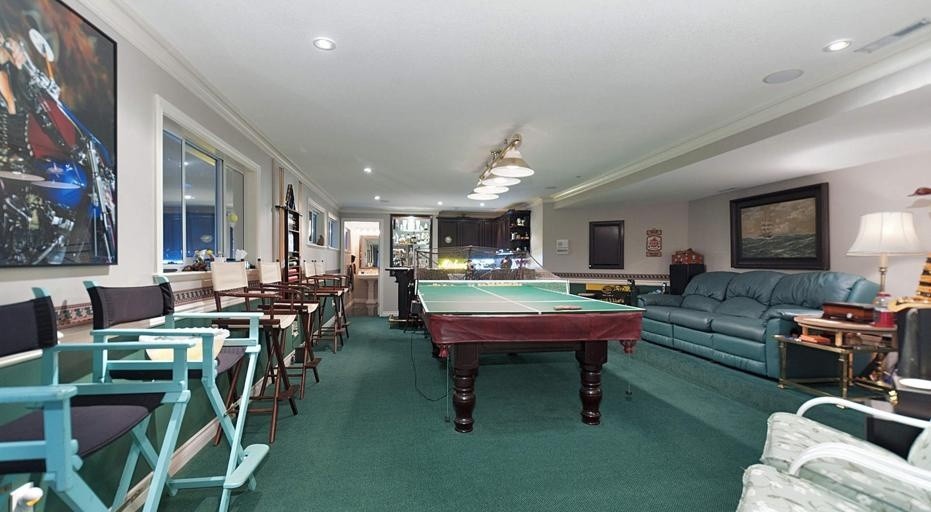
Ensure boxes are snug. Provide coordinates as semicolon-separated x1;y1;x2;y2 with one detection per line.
799;332;831;345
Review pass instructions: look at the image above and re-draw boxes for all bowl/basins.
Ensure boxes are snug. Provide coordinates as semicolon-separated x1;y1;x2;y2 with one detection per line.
138;326;230;363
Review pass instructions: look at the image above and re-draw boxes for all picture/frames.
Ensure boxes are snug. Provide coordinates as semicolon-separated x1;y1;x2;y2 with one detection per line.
0;0;119;268
730;181;832;271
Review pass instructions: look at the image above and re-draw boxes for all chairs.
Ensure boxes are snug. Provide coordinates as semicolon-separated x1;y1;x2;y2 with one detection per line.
85;275;271;512
257;256;349;396
760;395;931;512
1;287;199;512
734;447;931;512
211;259;300;446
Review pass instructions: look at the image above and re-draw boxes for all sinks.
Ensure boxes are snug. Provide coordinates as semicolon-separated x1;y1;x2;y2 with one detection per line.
357;270;378;280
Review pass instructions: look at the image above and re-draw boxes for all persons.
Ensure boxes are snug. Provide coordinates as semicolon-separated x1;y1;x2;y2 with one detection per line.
1;14;73;163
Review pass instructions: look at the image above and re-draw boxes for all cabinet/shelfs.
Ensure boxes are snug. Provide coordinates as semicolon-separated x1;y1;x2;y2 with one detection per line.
437;208;532;260
384;267;536;331
670;264;706;296
390;213;434;267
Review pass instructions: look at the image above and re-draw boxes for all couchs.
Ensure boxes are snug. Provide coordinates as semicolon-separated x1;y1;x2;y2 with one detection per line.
637;270;881;380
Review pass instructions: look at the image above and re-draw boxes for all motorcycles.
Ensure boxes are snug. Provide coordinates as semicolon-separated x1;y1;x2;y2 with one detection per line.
0;27;115;264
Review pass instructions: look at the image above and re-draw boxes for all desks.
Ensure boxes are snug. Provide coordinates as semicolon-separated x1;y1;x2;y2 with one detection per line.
772;335;900;410
792;315;899;350
412;278;649;433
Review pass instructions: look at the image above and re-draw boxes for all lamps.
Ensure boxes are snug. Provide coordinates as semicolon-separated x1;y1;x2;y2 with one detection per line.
468;132;536;202
846;209;927;329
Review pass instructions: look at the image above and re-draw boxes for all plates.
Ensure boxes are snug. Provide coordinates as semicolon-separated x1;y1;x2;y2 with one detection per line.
897;377;931;390
